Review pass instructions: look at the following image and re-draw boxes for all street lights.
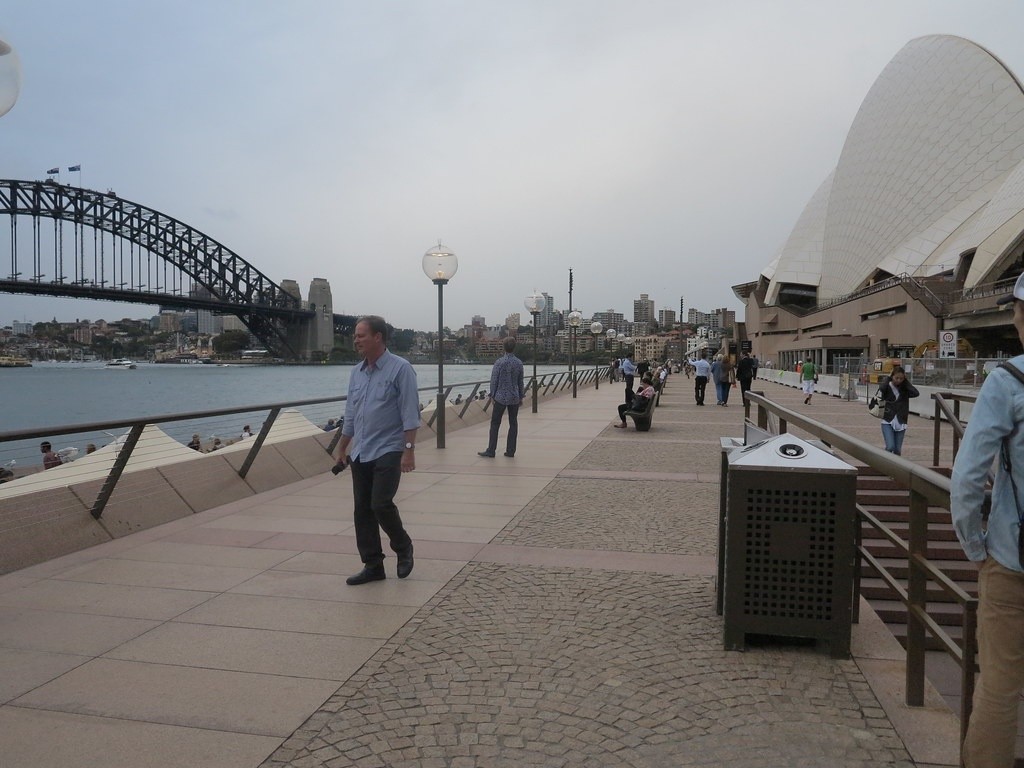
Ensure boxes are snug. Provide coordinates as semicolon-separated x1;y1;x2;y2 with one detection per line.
524;291;546;413
606;329;616;384
421;244;457;449
617;333;626;359
567;309;584;398
625;337;633;354
590;321;603;389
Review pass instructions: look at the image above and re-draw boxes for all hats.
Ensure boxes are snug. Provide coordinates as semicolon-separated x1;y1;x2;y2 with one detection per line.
798;361;803;364
996;273;1024;306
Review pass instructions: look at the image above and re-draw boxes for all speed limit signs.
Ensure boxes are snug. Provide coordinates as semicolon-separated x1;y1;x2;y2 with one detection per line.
943;333;954;342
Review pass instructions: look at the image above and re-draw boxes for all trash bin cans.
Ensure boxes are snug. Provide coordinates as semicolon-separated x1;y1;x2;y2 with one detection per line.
719;431;862;658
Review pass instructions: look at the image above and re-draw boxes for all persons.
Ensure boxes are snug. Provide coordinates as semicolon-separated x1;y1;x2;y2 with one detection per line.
950;271;1024;768
798;357;818;405
736;351;772;406
337;315;421;585
188;434;225;452
635;358;681;393
685;353;735;406
477;337;525;457
474;389;486;400
456;394;462;405
0;468;14;484
41;441;61;470
606;356;628;382
879;367;920;455
241;425;253;439
614;379;654;428
324;416;343;431
623;354;635;390
87;444;96;454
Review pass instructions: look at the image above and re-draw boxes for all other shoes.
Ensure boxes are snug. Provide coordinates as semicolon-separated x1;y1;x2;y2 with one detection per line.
808;403;811;405
504;451;514;457
700;402;704;406
717;400;721;405
804;398;809;404
697;401;700;405
614;424;617;427
722;402;727;407
618;423;627;428
478;449;495;457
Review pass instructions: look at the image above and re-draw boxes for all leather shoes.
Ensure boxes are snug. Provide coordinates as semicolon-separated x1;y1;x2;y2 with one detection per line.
397;544;414;578
346;566;386;585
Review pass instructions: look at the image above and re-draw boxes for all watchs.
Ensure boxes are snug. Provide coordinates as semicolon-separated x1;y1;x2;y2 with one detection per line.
404;442;415;448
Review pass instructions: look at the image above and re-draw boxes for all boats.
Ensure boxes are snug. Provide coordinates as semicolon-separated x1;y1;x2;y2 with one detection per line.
225;364;230;367
105;357;132;369
0;356;33;367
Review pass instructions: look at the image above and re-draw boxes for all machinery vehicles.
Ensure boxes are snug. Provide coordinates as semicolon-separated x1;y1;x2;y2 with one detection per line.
868;338;939;384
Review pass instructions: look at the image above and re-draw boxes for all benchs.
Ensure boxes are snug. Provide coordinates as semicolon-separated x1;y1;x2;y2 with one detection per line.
622;373;667;432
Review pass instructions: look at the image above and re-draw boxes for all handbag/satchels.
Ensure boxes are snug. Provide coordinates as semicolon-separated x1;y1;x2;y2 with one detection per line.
868;378;888;418
814;379;817;384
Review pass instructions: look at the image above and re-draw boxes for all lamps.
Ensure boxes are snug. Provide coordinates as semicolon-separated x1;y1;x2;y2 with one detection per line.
948;313;951;318
972;309;977;314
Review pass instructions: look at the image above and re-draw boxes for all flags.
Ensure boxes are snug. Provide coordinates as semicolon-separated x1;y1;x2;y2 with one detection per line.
68;165;80;171
47;168;59;174
